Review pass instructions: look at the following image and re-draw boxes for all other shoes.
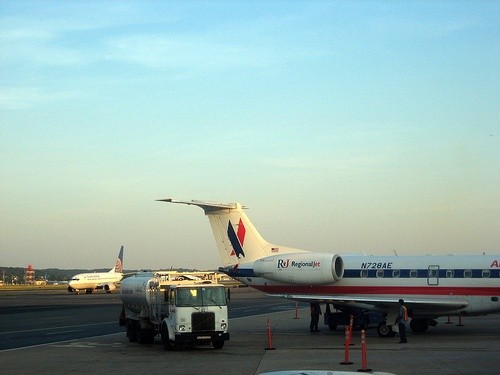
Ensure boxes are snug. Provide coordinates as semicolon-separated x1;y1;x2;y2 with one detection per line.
315;329;320;332
310;331;315;333
398;339;407;343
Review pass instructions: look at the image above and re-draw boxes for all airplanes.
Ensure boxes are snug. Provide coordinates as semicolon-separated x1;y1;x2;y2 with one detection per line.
155;200;500;339
68;246;127;295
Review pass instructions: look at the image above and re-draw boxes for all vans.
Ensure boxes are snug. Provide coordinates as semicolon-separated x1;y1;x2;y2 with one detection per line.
323;302;388;330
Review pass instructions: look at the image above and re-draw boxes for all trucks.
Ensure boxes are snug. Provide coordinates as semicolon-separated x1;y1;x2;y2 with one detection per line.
119;271;229;350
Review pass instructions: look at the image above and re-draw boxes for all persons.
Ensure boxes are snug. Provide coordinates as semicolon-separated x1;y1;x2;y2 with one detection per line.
309;302;323;333
394;299;408;344
